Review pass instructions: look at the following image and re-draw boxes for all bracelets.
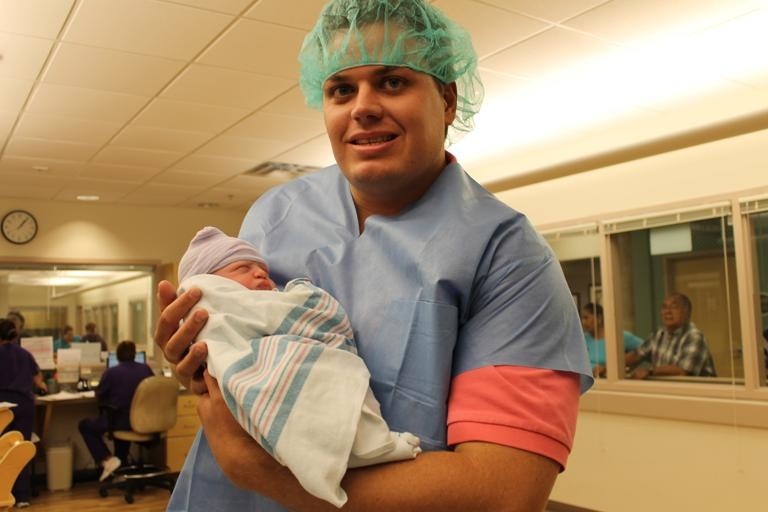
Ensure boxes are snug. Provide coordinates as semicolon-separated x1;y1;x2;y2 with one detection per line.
648;369;653;376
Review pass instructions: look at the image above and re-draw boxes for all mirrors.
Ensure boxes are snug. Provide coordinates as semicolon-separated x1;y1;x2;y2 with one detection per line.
0;256;157;382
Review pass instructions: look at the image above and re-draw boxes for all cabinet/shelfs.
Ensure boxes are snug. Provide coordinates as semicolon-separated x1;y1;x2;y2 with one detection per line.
160;388;207;477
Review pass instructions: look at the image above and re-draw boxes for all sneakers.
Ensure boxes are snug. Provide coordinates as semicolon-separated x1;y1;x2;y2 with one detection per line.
99;456;121;482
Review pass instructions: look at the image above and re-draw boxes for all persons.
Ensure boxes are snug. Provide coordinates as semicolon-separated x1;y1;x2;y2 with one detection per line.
155;0;595;512
80;324;107;351
178;225;422;509
6;310;25;347
54;326;75;350
581;302;646;369
78;340;154;482
0;319;49;508
593;293;716;380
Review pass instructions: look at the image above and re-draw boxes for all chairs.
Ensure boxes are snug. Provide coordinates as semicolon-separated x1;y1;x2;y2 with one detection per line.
98;374;180;505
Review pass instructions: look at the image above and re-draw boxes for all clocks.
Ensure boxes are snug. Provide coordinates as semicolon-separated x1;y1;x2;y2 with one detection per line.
0;208;38;244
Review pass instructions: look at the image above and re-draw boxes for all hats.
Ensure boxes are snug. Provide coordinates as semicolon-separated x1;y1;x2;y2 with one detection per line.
178;226;266;280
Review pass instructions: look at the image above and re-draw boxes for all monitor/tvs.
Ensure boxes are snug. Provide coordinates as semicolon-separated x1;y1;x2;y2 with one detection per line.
106;351;146;369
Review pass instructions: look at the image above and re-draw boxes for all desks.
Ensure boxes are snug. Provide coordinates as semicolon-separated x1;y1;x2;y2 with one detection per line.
31;385;202;474
